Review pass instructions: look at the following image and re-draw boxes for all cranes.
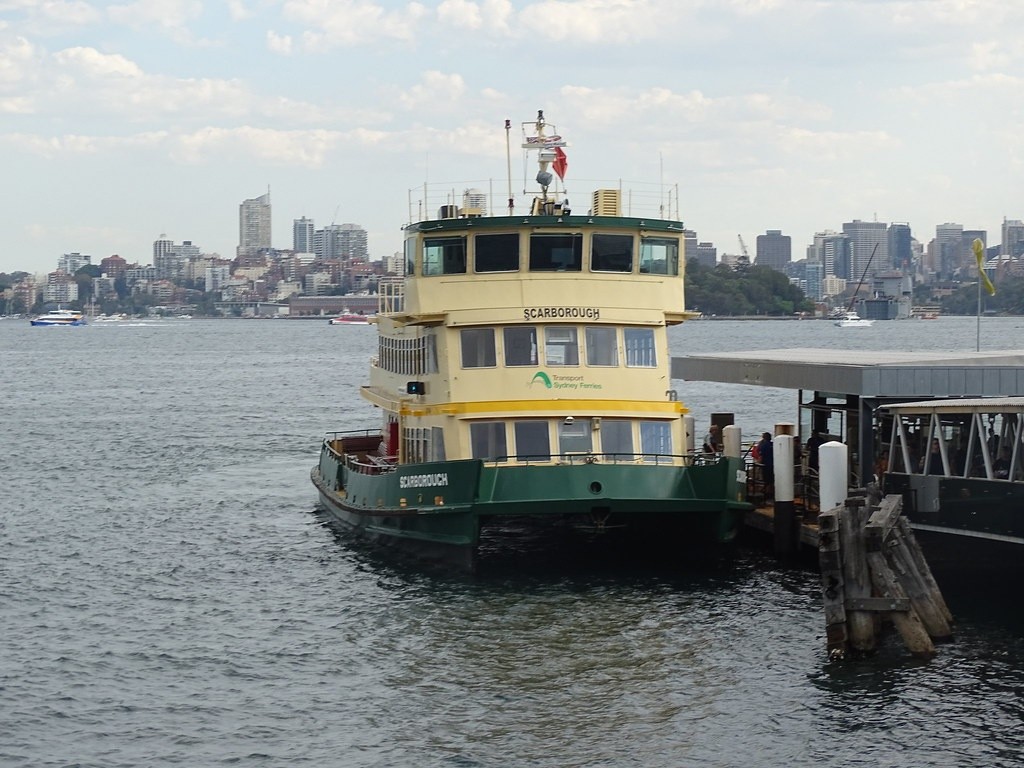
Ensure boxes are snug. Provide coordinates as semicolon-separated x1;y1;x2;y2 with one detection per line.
737;233;748;256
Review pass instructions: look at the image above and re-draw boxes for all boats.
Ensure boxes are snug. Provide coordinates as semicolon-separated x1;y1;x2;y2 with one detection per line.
328;307;379;325
29;310;88;326
834;312;872;327
307;110;758;572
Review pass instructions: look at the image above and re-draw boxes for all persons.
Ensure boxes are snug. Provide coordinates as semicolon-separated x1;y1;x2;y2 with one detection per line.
703;425;718;456
755;423;1011;500
